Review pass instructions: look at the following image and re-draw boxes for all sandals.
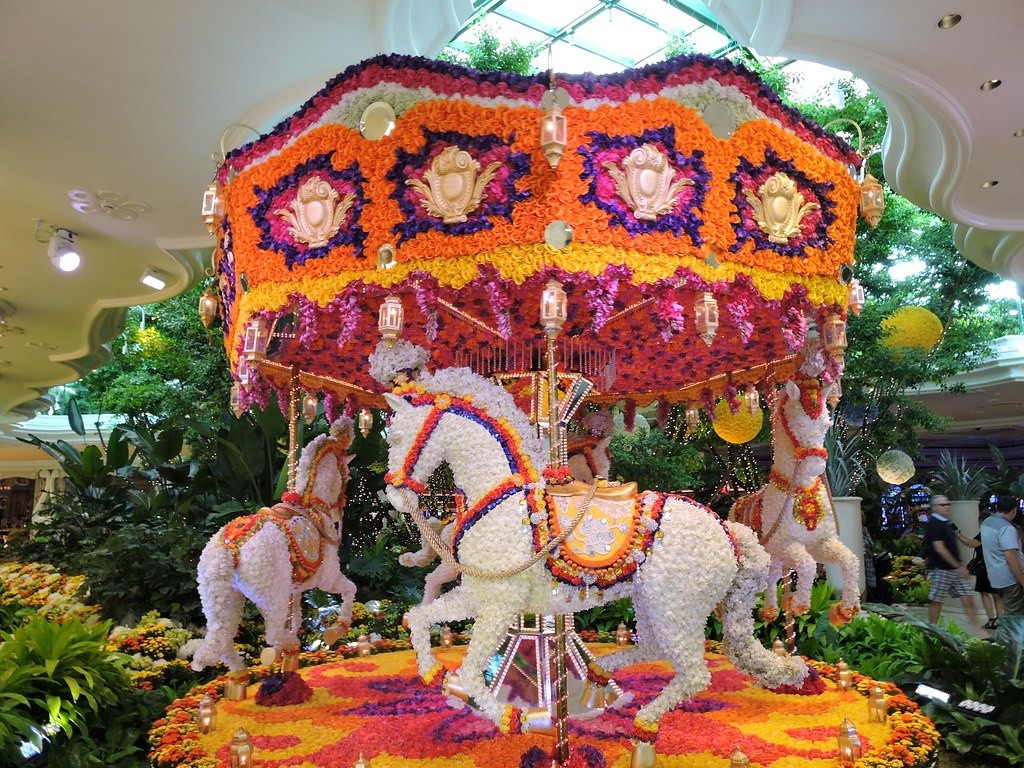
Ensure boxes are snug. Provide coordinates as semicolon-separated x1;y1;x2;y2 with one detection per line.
982;617;998;629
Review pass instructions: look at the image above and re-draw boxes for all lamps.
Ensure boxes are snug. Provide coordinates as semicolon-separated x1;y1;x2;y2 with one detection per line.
744;386;759;418
692;289;721;347
540;279;568;345
139;267;168;289
836;720;863;761
357;409;374;438
616;622;627;647
728;748;750;768
820;310;848;367
684;403;698;431
229;724;254;768
834;656;852;690
865;684;888;722
229;380;247;421
199;271;218;327
31;219;82;272
439;625;453;648
201;153;227;238
847;276;866;317
773;637;786;656
197;692;217;734
859;155;886;228
357;633;370;657
541;81;568;170
376;291;405;350
244;310;269;372
820;377;845;411
353;752;371;768
302;390;319;427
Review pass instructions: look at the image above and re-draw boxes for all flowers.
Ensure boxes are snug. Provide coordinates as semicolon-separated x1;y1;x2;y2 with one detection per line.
398;435;622;602
872;305;946;364
711;396;764;446
877;450;918;484
884;553;927;591
147;633;941;768
0;559;420;686
187;418;357;704
371;340;809;768
205;54;871;437
725;347;864;626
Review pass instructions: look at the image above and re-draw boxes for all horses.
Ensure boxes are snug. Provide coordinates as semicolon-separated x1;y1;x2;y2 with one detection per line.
189;364;863;751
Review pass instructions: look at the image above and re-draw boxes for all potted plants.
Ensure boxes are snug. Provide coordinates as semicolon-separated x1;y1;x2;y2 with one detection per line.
927;449;1003;614
825;415;875;599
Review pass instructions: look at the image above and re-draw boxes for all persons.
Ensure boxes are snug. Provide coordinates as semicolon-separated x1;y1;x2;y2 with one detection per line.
953;512;1002;629
979;495;1024;682
925;495;983;629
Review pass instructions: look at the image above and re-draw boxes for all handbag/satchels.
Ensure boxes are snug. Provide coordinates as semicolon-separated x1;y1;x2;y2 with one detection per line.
966;559;985;576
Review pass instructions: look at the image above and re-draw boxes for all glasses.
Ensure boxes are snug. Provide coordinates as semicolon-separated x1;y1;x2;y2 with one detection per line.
933;503;951;506
